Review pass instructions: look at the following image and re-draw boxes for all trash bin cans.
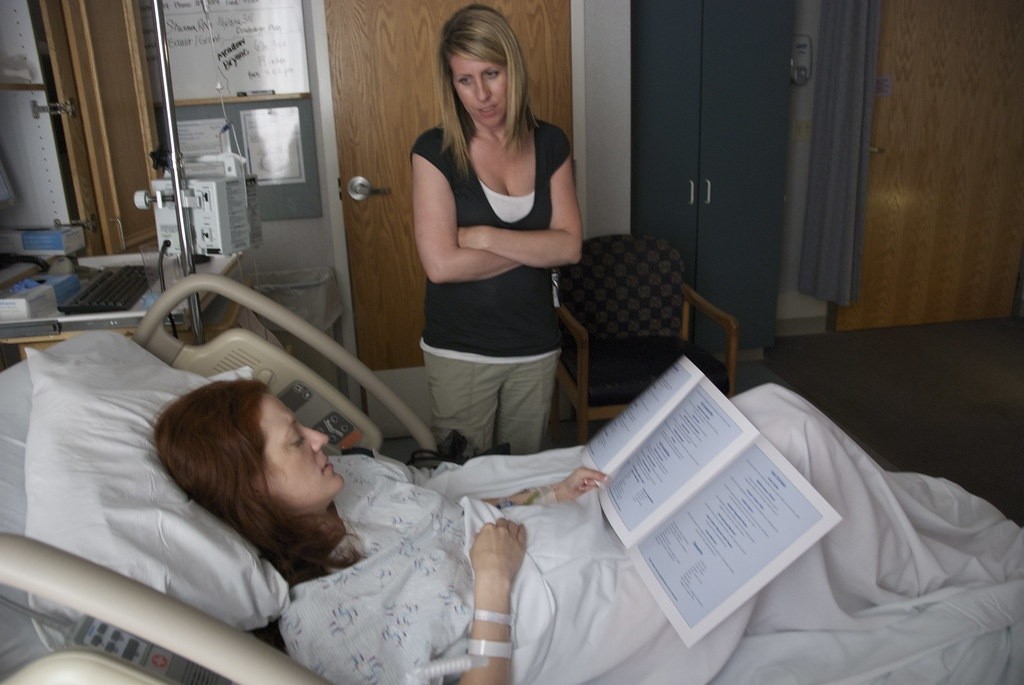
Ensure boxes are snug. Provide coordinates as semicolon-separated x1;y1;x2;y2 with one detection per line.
246;266;341;390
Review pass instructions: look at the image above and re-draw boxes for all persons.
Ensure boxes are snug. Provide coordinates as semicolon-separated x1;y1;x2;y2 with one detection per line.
410;6;584;457
154;379;606;685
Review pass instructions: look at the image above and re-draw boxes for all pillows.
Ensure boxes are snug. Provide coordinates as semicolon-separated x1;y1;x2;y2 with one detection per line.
21;345;291;632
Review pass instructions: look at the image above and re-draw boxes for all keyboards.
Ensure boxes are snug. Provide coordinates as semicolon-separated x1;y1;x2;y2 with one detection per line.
58;263;160;313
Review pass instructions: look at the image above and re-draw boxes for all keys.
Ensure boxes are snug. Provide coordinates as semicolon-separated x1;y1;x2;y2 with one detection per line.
551;272;562;308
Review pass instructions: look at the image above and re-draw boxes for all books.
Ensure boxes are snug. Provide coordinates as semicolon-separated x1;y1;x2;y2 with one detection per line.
582;353;843;648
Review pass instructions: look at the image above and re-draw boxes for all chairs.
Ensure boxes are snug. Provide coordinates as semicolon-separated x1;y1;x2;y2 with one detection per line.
549;233;740;448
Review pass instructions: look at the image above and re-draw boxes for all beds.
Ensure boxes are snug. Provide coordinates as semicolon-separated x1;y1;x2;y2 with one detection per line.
0;269;1024;685
